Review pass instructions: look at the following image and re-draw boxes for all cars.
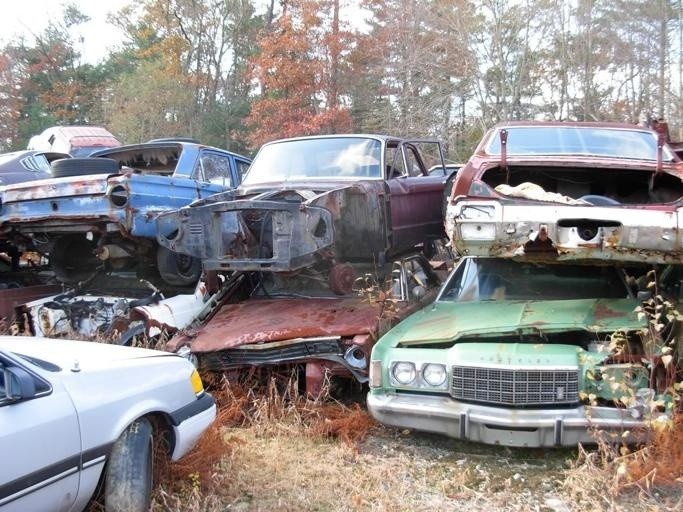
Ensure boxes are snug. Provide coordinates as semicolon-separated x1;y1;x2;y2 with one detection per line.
0;334;217;511
